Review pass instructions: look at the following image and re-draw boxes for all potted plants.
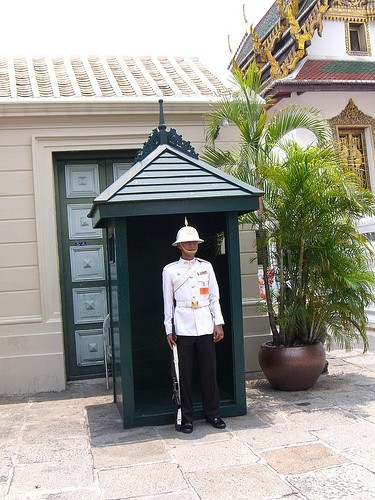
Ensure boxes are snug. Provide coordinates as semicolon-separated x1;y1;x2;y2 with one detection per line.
199;56;375;391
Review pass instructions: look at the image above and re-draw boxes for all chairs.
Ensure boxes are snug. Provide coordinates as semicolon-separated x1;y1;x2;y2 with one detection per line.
103;314;111;389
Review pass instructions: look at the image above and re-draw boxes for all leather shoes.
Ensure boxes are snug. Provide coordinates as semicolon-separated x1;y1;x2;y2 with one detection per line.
181;422;193;433
206;416;226;429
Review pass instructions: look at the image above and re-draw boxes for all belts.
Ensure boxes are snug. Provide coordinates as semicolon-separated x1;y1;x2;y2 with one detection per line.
176;300;209;308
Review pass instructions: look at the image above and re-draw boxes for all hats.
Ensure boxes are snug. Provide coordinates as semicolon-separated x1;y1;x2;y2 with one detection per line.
171;217;204;246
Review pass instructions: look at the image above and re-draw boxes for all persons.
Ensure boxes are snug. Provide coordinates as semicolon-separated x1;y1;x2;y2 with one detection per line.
162;216;227;434
